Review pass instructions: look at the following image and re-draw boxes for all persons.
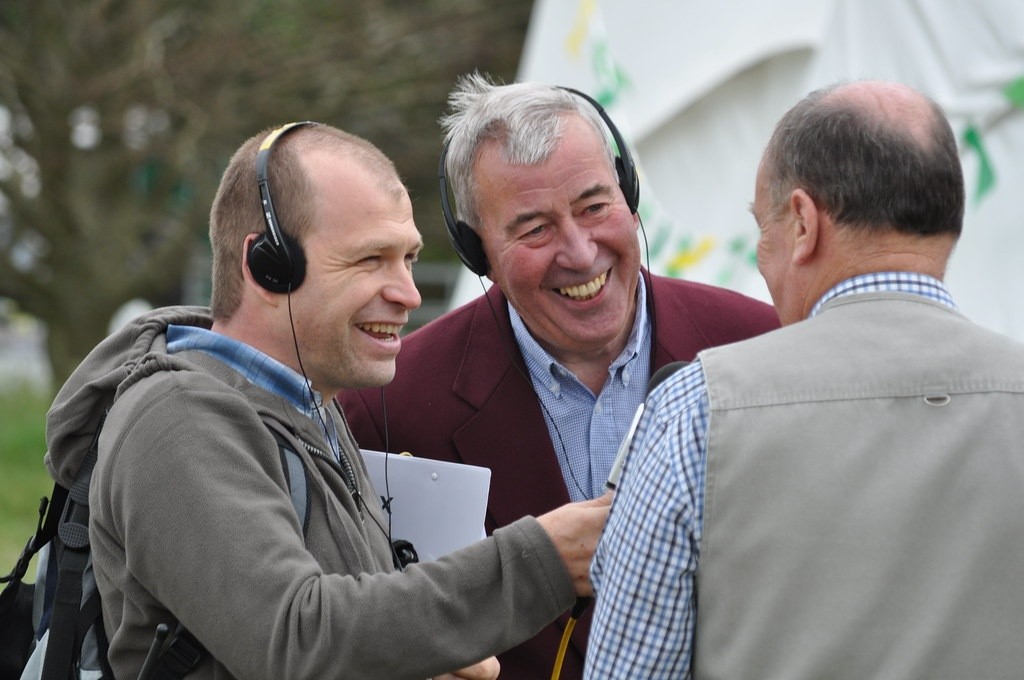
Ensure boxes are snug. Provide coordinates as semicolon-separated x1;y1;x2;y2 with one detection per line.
333;66;783;680
582;78;1023;679
41;118;614;680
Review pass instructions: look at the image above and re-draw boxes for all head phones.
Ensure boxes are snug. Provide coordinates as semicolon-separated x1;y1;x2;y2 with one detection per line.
439;87;639;276
247;121;316;295
